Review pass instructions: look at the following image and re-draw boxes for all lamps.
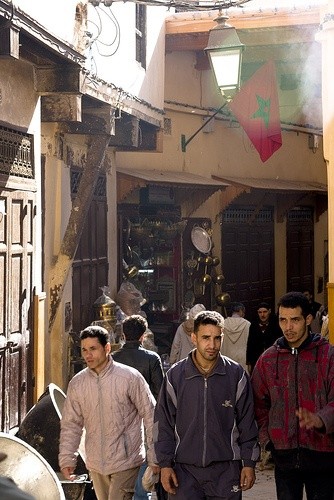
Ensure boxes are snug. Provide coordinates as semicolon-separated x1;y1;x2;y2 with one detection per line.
76;0;250;103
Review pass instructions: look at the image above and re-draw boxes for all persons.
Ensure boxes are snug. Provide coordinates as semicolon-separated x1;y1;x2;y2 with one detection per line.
153;295;261;500
58;315;164;500
246;290;334;500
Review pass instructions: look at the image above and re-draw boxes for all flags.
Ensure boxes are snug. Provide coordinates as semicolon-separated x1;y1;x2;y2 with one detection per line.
230;55;284;163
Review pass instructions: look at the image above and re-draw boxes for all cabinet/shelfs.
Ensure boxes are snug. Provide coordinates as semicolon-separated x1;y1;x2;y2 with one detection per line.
118;202;211;324
